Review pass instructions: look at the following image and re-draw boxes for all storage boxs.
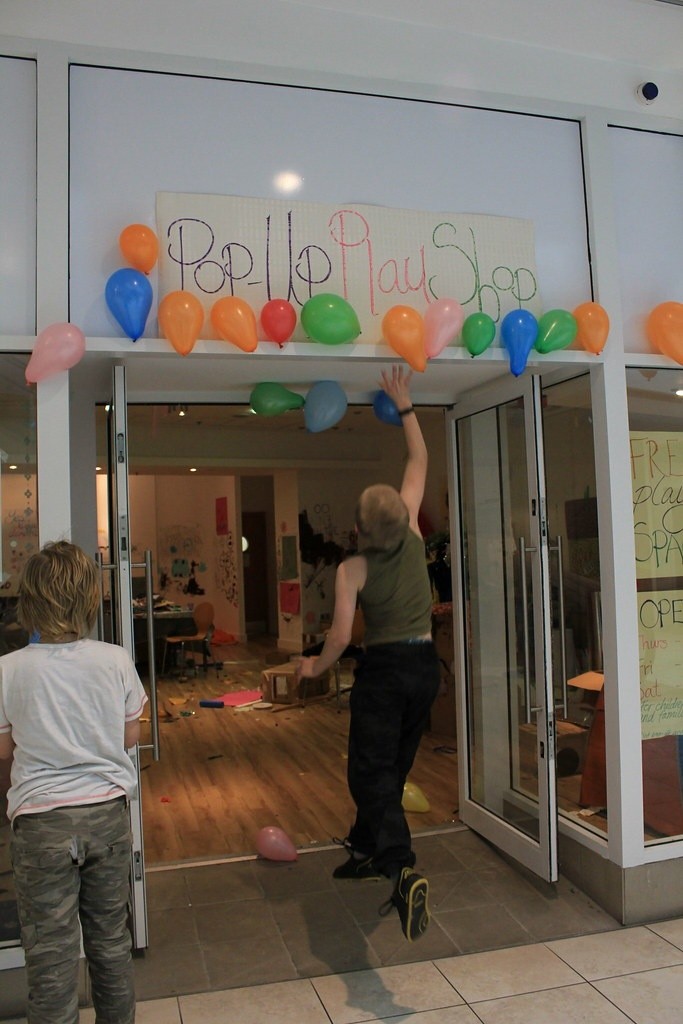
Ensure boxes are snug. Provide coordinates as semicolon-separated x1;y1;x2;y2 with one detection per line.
566;669;604;708
428;613;457;738
260;662;331;705
519;717;591;778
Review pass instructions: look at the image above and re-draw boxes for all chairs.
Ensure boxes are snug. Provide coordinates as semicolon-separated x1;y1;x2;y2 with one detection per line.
160;602;216;683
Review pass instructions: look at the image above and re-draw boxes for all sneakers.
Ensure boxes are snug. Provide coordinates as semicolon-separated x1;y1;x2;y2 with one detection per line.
331;835;383;884
379;867;430;944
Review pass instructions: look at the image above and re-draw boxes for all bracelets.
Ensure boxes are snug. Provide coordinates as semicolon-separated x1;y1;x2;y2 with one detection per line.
398;407;415;417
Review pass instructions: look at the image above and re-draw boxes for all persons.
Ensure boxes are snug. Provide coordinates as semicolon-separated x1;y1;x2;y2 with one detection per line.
0;542;149;1024
293;362;442;943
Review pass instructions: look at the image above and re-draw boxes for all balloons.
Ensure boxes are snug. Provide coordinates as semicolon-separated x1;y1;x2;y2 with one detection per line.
24;323;85;386
302;381;347;434
158;291;205;356
301;294;362;345
253;826;299;862
639;369;657;382
105;224;160;343
462;312;496;358
210;296;258;352
373;390;403;427
401;782;430;812
250;383;305;417
650;302;683;366
382;298;464;373
502;302;609;378
261;298;297;349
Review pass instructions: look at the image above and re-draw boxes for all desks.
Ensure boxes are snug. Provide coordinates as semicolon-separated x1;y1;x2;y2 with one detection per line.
133;610;215;679
